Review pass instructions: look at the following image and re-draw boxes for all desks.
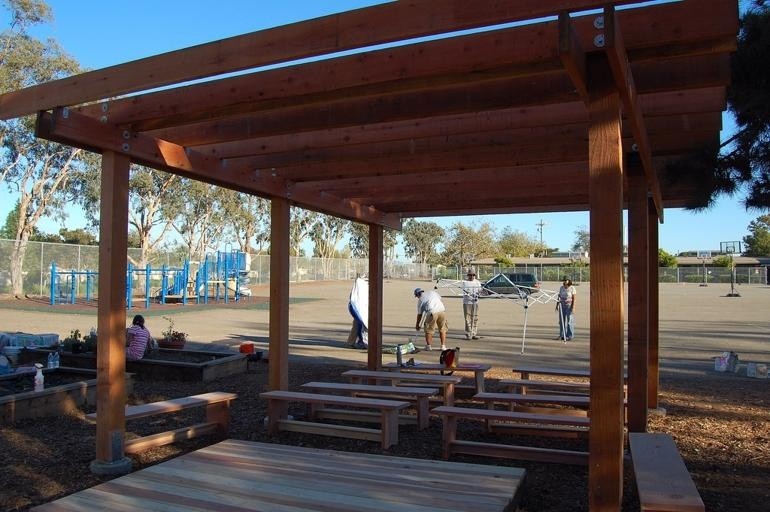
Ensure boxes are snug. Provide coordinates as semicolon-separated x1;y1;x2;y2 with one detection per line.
536;220;547;247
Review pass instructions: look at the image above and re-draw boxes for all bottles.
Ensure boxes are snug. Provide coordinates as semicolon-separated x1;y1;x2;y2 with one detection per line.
414;288;424;296
562;275;571;280
467;270;476;276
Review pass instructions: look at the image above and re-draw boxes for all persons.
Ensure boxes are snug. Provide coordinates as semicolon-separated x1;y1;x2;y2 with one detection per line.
344;273;370;349
462;268;482;340
414;288;449;351
552;276;577;341
126;314;154;362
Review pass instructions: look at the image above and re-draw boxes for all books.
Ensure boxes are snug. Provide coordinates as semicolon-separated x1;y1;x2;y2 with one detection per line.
747;363;768;379
714;356;736;372
9;332;58;350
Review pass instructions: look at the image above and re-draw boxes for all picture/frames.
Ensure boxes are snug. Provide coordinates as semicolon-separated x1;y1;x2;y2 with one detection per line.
24;439;525;512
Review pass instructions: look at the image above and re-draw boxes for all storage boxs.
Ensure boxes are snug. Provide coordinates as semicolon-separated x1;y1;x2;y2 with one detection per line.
86;391;238;456
627;432;706;512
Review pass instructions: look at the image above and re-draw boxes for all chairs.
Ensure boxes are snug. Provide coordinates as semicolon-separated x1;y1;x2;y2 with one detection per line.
557;335;572;341
467;335;479;341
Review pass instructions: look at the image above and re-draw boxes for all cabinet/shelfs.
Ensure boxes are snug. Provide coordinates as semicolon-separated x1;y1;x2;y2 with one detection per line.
48;352;60;369
748;363;768;379
397;347;401;366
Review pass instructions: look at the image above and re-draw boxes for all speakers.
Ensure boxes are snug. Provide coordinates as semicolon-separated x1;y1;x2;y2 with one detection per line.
424;344;431;350
440;344;447;351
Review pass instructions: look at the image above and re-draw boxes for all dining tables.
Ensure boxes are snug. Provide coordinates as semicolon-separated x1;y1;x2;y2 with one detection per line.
84;328;97;351
60;330;81;352
157;315;189;348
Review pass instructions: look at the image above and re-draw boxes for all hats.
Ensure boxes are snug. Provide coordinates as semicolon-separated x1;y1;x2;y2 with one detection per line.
482;273;540;298
